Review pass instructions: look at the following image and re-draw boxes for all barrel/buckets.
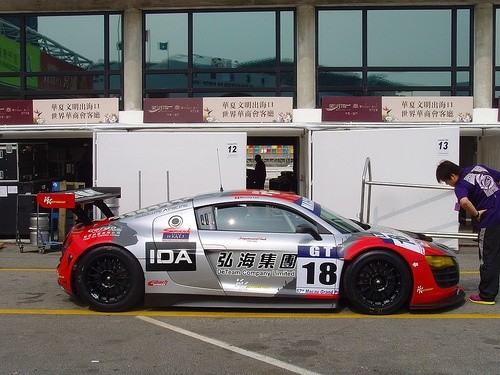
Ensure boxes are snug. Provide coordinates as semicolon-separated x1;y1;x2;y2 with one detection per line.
29;213;49;228
29;227;49;247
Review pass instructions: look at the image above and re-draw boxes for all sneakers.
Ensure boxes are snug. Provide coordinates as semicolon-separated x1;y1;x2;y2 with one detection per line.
469;295;495;305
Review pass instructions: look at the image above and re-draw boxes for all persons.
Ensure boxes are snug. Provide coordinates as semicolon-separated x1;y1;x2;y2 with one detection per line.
434;158;500;306
251;153;267;190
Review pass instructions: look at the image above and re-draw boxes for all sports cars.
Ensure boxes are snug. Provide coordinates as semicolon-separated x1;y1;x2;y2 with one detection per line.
35;188;466;316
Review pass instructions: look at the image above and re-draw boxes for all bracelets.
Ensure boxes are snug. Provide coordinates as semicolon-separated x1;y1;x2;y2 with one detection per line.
470;212;480;220
